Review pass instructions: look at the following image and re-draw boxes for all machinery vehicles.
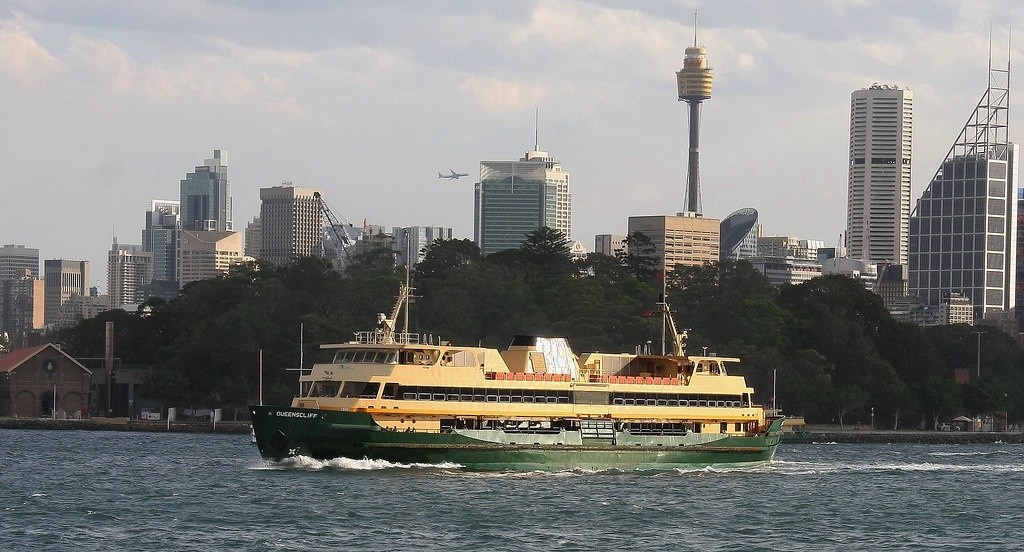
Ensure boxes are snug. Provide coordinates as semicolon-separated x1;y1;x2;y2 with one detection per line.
311;191;379;323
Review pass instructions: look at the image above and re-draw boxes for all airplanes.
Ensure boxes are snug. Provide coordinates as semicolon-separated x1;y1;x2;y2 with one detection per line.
438;169;470;180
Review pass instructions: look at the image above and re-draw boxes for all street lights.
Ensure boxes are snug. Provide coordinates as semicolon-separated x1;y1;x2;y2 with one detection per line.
871;407;875;431
1003;393;1008;431
969;331;989;377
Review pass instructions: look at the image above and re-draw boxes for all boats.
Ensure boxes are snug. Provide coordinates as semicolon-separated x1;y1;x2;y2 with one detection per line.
247;235;788;465
